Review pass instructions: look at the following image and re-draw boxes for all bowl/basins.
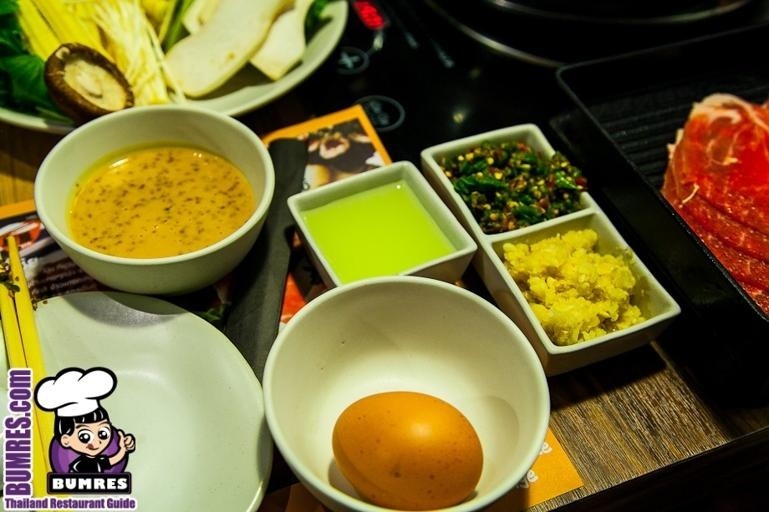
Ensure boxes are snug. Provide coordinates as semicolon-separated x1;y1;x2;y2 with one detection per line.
35;104;682;512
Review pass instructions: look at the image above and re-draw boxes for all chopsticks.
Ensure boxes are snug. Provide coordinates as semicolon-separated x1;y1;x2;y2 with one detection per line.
0;237;57;505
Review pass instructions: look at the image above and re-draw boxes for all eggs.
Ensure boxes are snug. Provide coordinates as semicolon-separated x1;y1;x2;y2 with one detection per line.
332;391;483;512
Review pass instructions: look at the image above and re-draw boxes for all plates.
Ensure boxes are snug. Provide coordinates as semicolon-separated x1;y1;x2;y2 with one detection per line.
0;289;274;512
0;1;352;133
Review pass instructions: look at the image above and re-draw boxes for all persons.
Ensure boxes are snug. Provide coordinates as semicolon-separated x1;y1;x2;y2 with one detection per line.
54;407;136;474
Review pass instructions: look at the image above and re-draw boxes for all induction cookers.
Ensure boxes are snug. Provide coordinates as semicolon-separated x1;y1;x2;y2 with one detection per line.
241;0;553;161
